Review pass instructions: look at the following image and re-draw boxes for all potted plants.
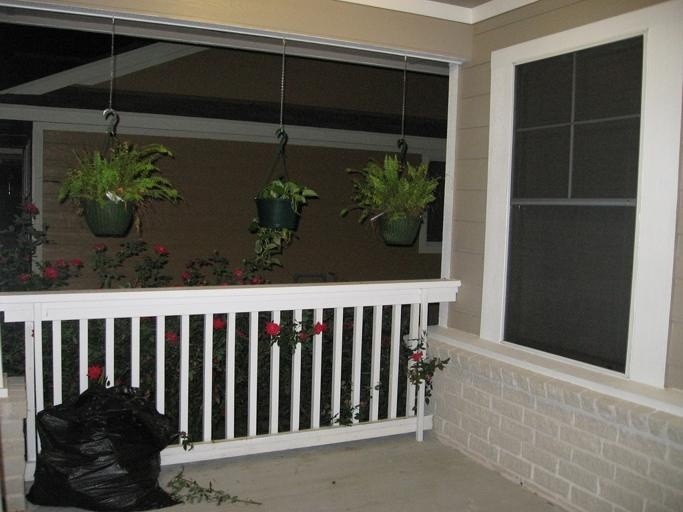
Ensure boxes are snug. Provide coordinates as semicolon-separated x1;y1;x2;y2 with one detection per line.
342;140;441;244
255;180;319;267
53;141;183;236
